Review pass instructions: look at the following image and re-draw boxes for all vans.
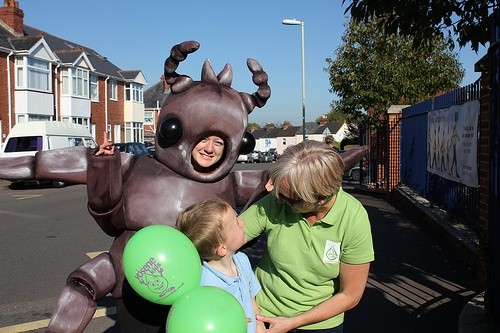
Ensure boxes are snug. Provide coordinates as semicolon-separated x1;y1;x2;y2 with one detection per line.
0;119;99;188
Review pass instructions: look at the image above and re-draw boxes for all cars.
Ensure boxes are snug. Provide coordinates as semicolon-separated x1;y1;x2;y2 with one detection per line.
236;148;281;164
110;141;148;159
339;150;369;182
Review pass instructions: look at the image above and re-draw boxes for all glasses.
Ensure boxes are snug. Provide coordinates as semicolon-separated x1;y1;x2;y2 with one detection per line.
278;193;303;208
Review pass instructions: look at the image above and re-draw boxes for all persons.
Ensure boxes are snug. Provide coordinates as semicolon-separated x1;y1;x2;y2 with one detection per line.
0;41;375;333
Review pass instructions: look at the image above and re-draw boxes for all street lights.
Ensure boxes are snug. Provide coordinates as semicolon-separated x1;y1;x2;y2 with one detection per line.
281;18;306;141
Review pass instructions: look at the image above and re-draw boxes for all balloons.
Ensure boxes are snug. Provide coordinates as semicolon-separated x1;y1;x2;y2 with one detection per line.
122;224;202;305
165;286;247;333
